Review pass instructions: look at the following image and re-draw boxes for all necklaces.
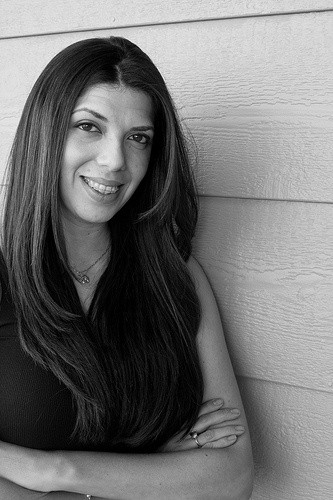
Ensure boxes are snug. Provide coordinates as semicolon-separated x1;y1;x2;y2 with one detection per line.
65;245;110;285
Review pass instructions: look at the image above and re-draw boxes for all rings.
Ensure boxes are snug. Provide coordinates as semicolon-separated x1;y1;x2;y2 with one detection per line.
189;430;203;449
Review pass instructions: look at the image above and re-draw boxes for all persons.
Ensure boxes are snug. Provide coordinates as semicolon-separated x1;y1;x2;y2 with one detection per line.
1;36;255;499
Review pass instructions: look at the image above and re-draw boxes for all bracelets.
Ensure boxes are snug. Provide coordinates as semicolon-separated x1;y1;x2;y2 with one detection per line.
86;494;92;500
